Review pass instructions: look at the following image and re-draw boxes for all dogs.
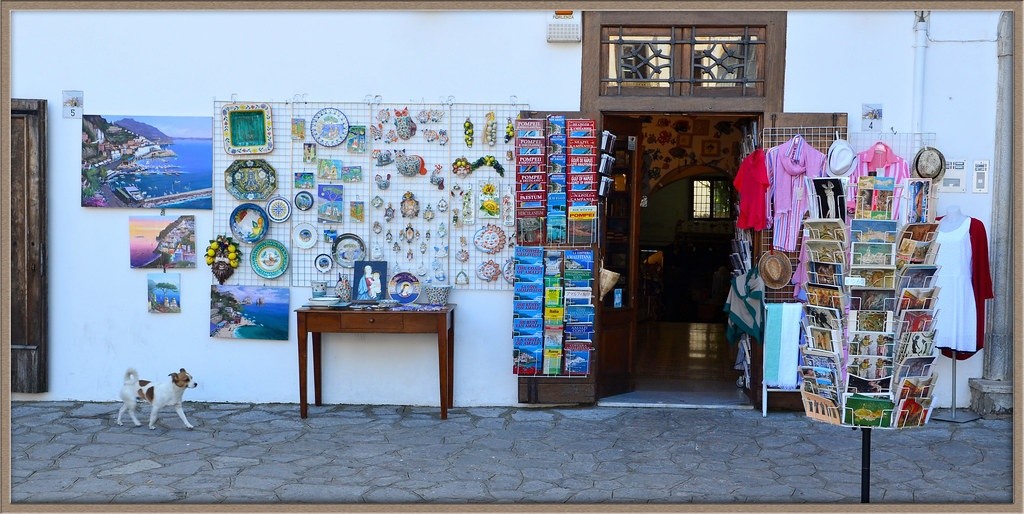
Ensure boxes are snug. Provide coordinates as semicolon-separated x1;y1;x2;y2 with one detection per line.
116;367;198;430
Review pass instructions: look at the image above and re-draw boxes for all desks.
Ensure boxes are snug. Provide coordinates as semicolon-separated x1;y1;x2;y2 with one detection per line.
293;303;457;419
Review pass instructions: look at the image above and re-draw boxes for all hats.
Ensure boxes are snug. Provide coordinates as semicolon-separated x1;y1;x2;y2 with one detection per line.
758;249;792;289
912;146;947;184
826;138;857;178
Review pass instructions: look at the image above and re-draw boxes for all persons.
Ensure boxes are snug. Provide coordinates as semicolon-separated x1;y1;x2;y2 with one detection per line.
929;205;995;360
645;197;727;321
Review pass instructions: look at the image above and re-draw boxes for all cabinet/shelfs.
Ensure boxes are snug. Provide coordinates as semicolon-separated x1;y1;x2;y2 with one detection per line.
596;143;639;399
